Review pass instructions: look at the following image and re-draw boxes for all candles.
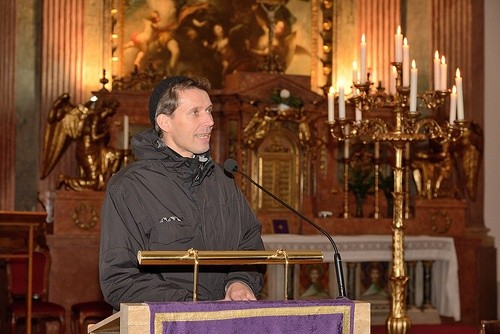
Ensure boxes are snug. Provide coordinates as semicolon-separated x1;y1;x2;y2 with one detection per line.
395;25;403;62
440;55;448;92
434;50;441;91
402;37;409;87
450;85;458;125
455;68;464;120
410;60;418;112
355;89;362;121
328;87;334;121
360;34;366;84
392;66;397;94
352;61;357;94
339;78;346;119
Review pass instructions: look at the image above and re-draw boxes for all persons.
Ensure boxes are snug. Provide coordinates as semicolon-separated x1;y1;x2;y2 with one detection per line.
98;76;267;312
362;262;389;298
301;264;330;299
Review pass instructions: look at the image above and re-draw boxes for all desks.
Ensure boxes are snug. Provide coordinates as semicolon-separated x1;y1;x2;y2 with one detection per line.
261;234;461;325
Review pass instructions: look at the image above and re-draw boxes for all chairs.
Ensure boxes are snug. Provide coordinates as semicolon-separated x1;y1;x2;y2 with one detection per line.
7;248;66;334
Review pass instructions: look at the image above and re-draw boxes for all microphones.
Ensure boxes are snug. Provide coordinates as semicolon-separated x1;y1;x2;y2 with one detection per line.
224;159;347;298
